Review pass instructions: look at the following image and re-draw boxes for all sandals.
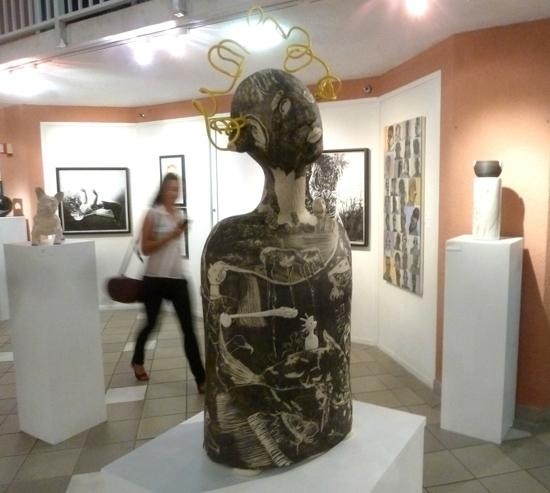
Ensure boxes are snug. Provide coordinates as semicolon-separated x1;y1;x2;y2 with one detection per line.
130;361;149;381
197;385;205;394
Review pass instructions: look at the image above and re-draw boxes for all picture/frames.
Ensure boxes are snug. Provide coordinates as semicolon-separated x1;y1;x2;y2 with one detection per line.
159;155;188;259
55;167;130;235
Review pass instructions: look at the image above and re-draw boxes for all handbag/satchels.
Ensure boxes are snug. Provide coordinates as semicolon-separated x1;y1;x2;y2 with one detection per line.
107;277;143;303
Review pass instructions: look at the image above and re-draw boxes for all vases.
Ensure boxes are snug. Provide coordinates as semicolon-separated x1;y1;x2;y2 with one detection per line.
0;181;12;217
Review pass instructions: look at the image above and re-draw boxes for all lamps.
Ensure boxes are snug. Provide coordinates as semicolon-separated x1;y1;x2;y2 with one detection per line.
170;1;186;18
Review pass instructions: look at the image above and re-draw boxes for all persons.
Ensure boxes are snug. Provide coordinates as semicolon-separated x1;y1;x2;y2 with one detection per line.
131;174;206;394
201;69;353;468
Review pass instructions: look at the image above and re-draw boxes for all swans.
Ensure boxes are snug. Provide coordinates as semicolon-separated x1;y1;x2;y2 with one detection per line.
79;188;103;215
